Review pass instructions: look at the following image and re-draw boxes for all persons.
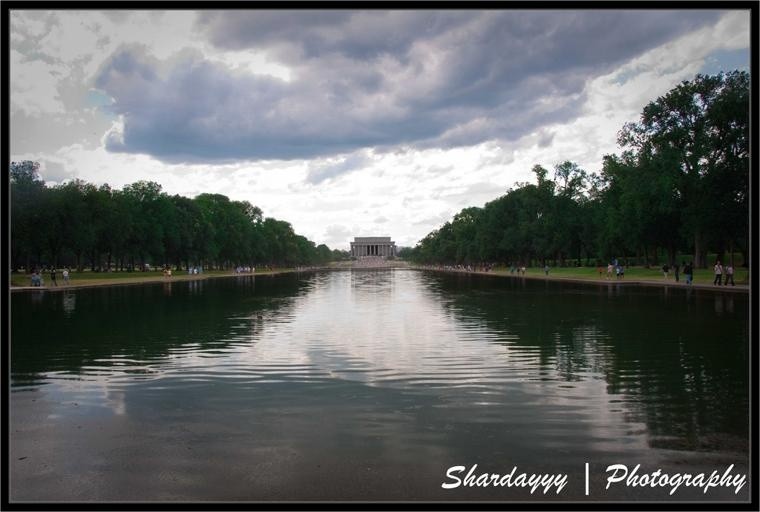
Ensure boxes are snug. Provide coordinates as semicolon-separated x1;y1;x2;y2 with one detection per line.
662;261;693;284
449;262;494;272
29;264;69;286
188;264;198;275
510;264;525;276
607;261;614;279
620;265;624;280
713;260;722;286
597;261;603;278
232;262;256;273
162;263;171;278
616;266;620;280
545;263;548;275
724;260;736;287
265;264;273;272
